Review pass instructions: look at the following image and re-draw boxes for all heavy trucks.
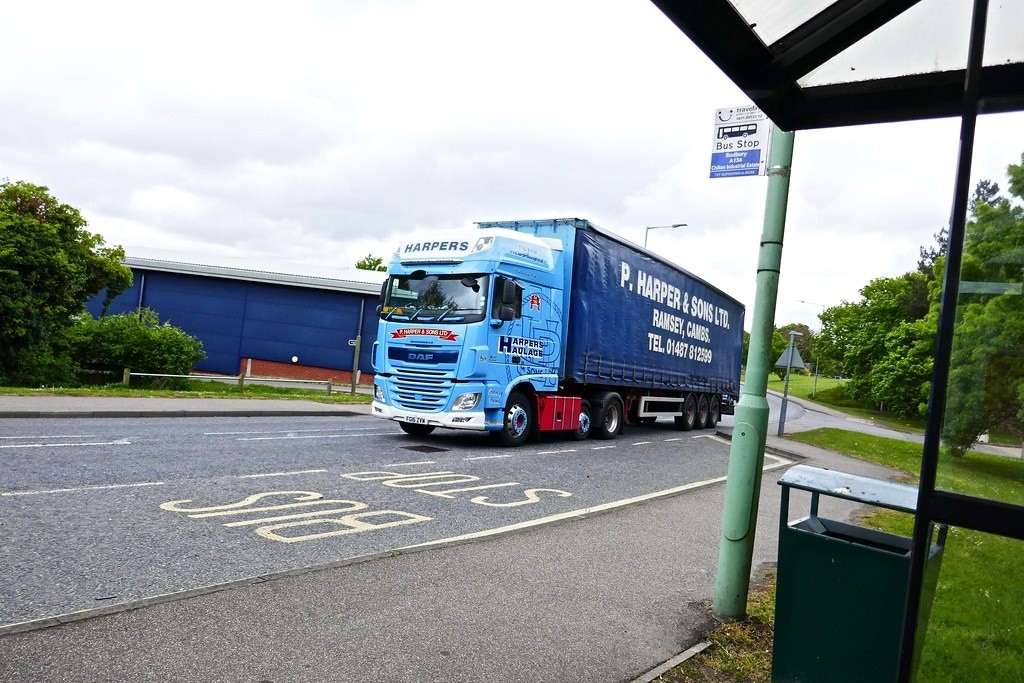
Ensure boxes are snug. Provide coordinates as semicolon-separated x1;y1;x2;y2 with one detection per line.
370;214;746;448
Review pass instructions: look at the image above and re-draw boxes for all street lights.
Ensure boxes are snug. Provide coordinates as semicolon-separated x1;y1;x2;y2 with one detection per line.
801;300;826;338
642;222;690;248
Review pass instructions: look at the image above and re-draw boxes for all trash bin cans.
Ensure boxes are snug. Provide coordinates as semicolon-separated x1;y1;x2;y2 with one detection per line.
769;458;951;683
